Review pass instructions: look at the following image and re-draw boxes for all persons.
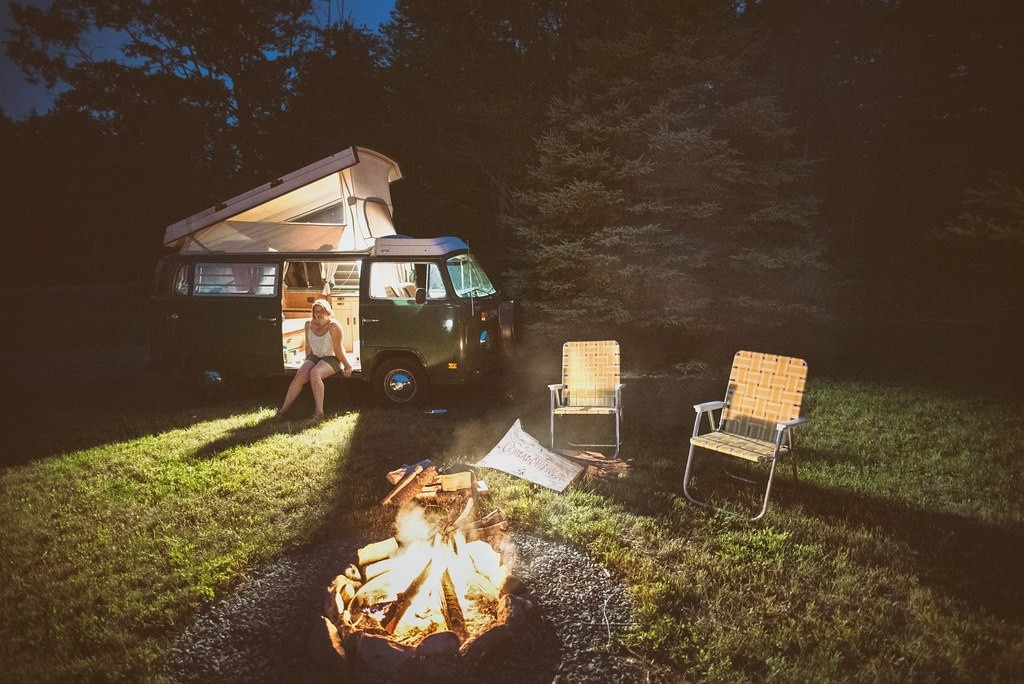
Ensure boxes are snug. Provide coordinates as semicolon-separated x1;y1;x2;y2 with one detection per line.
274;298;353;421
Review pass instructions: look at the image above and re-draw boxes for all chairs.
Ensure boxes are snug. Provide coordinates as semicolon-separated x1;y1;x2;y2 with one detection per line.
548;340;627;459
386;282;419;298
684;350;809;521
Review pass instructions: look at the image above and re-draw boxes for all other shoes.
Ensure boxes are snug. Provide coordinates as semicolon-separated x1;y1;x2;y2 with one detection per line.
302;412;325;425
275;409;288;422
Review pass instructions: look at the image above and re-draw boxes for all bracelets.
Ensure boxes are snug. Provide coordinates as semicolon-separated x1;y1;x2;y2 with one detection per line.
345;366;351;370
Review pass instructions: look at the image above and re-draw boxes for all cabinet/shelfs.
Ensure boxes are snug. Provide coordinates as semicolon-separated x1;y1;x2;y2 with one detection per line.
330;293;360;352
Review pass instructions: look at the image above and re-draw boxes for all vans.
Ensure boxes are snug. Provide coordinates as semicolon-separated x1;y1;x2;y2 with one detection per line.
154;147;518;403
